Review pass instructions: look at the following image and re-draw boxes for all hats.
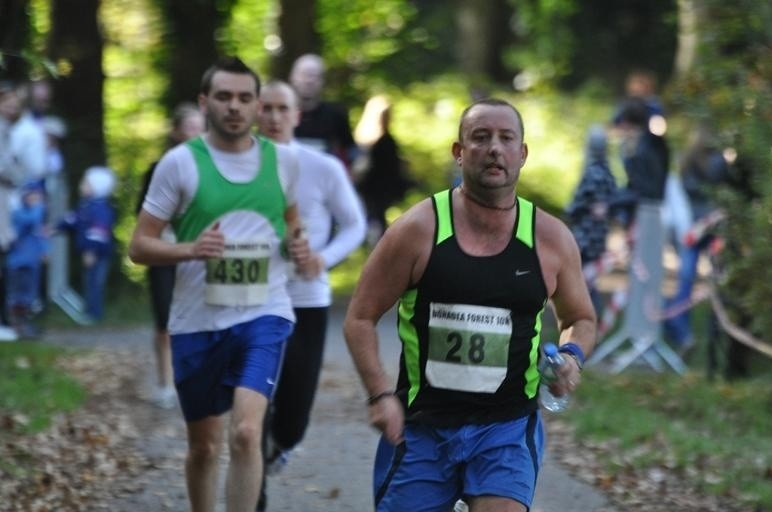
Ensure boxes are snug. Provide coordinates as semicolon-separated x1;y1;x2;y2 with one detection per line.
84;167;114;198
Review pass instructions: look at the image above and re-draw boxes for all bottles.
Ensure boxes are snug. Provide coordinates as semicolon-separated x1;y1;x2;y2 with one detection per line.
533;342;570;416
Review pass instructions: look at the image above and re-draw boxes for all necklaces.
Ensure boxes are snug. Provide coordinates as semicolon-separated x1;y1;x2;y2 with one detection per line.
459;185;517;210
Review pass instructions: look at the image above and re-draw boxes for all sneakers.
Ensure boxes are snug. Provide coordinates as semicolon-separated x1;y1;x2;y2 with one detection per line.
150;386;177;409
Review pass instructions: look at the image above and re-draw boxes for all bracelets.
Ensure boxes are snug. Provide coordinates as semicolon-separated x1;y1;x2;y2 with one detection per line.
556;342;585;372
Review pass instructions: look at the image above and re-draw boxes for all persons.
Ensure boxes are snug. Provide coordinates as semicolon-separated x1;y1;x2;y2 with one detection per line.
128;57;310;512
0;81;113;342
287;56;360;171
563;74;754;263
342;98;598;511
350;94;407;248
258;79;367;476
134;102;207;406
